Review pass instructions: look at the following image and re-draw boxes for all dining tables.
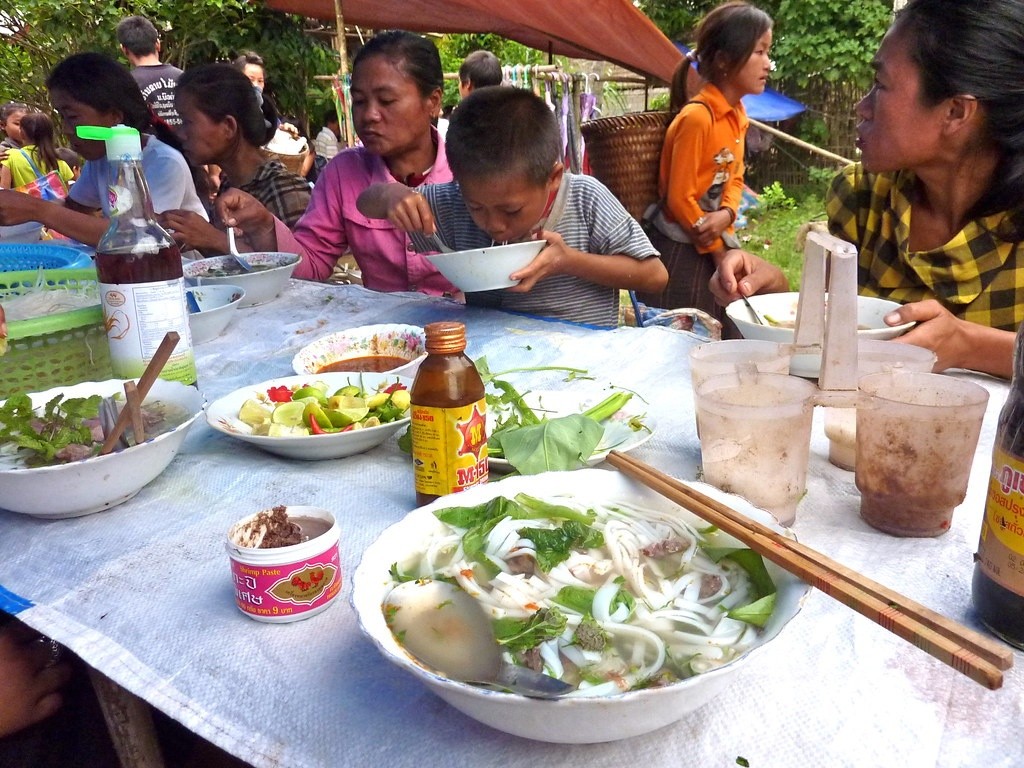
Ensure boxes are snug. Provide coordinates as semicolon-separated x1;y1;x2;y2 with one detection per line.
1;272;1024;766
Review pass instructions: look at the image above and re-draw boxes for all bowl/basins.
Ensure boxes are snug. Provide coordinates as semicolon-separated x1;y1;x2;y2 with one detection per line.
424;239;552;293
228;507;344;623
207;372;423;460
292;323;428;384
0;377;206;519
183;283;245;343
182;252;303;309
350;469;811;745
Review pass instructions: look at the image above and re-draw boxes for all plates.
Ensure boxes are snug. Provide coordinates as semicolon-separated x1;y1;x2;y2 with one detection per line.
452;365;658;473
725;288;916;379
1;219;45;246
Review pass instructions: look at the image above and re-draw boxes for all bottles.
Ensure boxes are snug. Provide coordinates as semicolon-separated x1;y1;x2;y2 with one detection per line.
74;123;199;395
968;319;1024;652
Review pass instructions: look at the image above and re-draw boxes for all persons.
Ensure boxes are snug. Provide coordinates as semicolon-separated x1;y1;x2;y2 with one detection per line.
0;15;345;253
639;2;774;341
711;0;1024;384
355;84;669;326
212;29;467;306
458;49;505;98
0;614;71;735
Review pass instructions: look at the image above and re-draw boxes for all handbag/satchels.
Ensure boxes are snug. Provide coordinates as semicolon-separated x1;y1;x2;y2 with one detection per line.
8;149;69;205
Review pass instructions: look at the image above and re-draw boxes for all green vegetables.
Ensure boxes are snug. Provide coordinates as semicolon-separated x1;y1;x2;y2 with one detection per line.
386;492;780;685
0;389;123;464
396;355;655;483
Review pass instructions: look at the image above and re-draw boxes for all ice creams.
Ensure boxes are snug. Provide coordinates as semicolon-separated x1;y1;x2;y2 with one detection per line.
254;507;331;548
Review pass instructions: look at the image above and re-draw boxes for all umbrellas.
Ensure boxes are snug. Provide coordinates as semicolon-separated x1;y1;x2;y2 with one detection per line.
511;63;601;175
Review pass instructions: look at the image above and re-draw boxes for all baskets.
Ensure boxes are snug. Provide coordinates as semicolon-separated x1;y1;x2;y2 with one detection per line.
0;269;112;401
0;243;93;298
579;112;675;225
259;129;310;176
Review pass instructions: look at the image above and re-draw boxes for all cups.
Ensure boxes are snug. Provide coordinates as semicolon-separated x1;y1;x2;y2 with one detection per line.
409;321;490;511
690;337;792;449
694;373;815;529
854;370;990;539
819;340;938;474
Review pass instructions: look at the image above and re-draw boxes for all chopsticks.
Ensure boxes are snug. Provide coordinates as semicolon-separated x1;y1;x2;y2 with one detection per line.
602;447;1018;692
98;330;180;456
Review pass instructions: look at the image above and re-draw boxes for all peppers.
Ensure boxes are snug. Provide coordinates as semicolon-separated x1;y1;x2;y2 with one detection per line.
308;414;353;434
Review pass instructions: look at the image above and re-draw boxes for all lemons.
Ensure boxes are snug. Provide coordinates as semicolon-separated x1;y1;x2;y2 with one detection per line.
235;383;413;437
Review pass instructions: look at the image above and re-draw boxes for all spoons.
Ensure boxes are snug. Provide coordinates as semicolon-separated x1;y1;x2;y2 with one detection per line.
224;218;252;271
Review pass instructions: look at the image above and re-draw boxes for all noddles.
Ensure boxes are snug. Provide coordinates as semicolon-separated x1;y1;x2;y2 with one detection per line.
192;264;280;287
384;491;782;701
491;239;508;247
